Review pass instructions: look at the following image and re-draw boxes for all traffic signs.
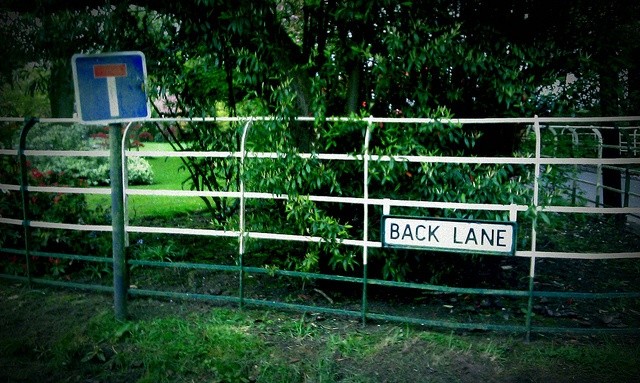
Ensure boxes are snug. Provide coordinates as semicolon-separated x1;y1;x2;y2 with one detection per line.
380;215;517;257
